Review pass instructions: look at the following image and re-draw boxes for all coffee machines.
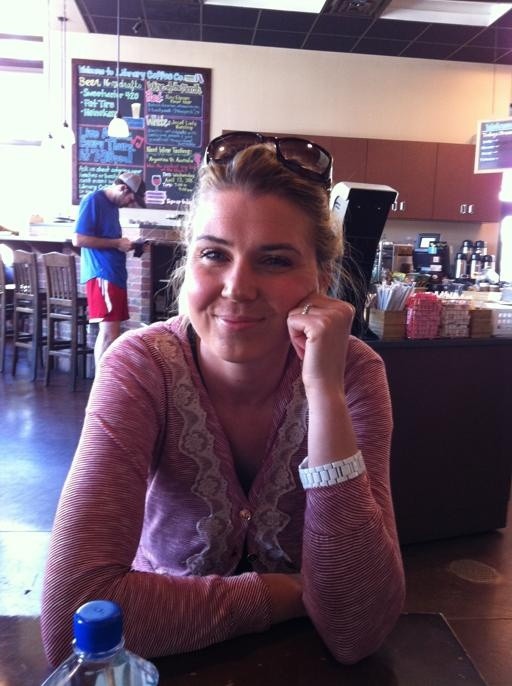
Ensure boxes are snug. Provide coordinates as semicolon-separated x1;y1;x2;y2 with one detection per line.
392;243;415;288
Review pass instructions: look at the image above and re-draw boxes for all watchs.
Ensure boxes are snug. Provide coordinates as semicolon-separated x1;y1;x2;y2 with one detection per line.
297;451;369;490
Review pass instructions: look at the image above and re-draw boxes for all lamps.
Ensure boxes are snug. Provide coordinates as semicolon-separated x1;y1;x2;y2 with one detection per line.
107;1;130;138
41;16;76;168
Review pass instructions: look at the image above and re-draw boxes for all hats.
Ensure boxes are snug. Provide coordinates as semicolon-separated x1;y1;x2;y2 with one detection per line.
117;171;147;208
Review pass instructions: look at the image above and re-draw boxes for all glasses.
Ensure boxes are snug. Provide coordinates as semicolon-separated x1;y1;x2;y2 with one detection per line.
201;130;334;200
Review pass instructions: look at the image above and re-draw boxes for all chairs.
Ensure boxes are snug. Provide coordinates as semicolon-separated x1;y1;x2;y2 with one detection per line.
9;250;39;382
42;251;93;393
0;259;15;373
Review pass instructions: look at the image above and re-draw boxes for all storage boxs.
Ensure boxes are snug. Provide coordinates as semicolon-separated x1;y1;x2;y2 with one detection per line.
369;307;406;340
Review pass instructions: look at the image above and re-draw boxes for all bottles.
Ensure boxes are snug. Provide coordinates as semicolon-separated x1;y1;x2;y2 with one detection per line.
42;599;162;685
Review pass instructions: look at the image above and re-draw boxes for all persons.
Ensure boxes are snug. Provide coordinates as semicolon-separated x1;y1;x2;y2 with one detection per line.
37;132;408;666
73;172;149;366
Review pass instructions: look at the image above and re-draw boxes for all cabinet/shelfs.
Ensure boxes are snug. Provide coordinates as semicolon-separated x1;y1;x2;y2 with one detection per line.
433;139;502;224
366;135;437;221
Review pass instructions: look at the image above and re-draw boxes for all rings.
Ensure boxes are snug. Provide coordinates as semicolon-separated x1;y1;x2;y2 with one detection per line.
297;303;313;318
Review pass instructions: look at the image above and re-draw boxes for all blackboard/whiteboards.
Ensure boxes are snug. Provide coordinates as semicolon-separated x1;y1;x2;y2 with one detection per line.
71;57;211;208
